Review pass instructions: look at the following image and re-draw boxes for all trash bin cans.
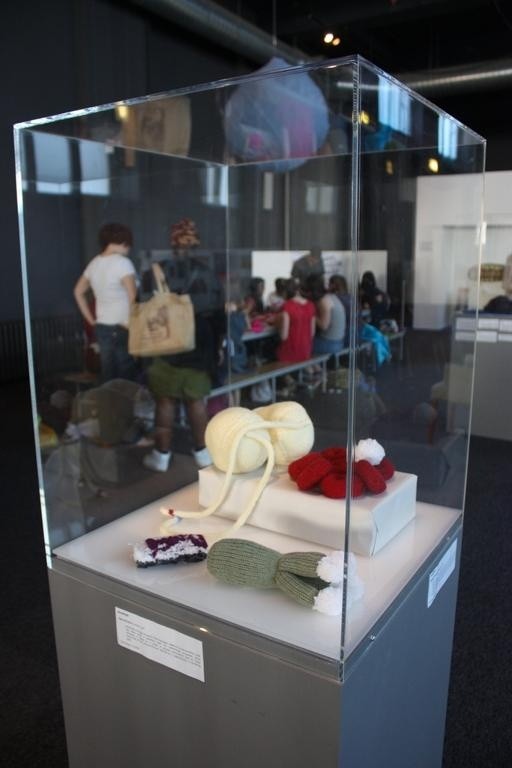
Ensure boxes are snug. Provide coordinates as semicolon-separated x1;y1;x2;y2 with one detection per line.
75;393;157;487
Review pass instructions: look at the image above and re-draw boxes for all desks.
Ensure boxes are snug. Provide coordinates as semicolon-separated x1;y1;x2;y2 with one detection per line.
214;307;289;345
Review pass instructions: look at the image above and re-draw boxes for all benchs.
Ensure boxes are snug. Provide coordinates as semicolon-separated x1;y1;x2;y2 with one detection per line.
177;329;407;407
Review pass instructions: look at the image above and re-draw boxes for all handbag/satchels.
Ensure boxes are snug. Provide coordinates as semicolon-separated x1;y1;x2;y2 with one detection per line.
127;289;196;358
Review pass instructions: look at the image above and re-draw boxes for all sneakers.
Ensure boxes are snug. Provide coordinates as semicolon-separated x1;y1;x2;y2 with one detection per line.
192;447;212;467
249;365;322;403
142;448;171;473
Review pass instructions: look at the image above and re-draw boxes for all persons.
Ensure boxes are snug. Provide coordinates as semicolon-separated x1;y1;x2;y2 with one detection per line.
73;219;137;380
206;241;394;421
137;217;227;474
